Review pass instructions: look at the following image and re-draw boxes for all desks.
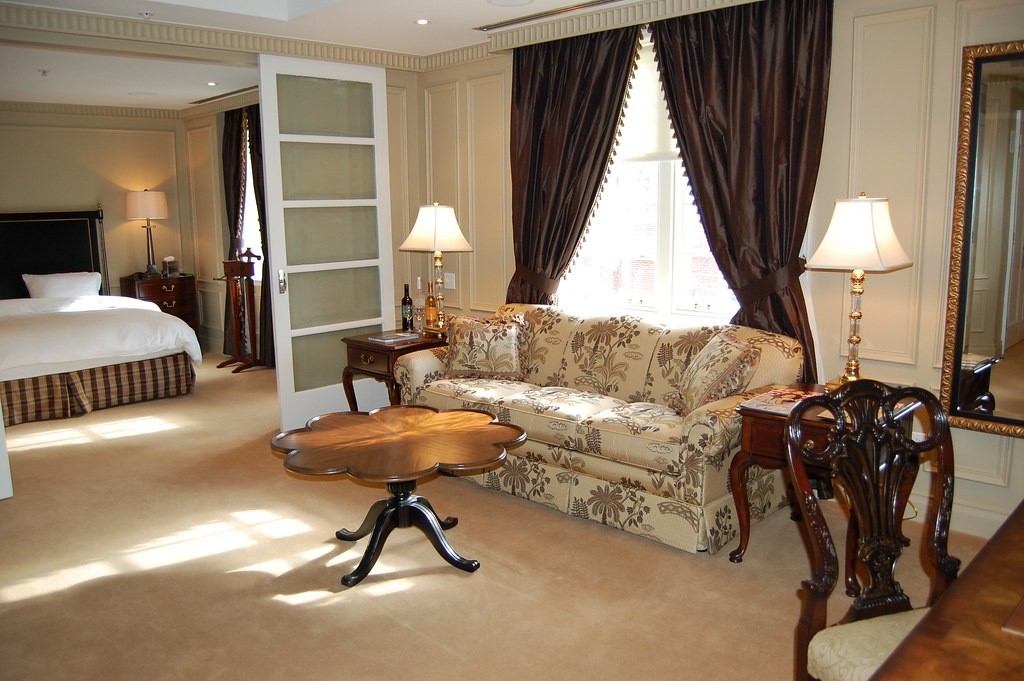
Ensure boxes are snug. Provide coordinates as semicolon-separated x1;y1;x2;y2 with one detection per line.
864;498;1023;681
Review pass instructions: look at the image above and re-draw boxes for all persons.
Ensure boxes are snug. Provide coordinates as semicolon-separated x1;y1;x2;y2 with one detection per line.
760;393;802;411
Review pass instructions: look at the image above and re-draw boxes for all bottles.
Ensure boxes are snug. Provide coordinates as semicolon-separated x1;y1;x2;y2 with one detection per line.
414;276;426;332
426;282;437;328
402;284;414;333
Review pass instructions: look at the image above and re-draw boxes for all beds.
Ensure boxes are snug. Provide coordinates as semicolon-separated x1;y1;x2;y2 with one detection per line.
0;202;204;431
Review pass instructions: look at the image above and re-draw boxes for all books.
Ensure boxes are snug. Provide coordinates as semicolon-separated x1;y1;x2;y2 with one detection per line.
368;333;419;343
826;377;845;390
740;387;825;417
961;354;991;370
817;399;923;424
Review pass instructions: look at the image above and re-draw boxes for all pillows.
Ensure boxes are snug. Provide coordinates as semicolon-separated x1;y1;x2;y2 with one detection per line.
445;312;528;381
21;272;103;300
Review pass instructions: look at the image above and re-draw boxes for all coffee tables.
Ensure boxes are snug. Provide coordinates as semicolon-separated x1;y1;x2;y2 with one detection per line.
268;402;528;589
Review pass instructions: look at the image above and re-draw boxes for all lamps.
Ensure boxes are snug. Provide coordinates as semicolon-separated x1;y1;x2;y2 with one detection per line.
125;189;170;280
396;200;474;340
804;191;914;394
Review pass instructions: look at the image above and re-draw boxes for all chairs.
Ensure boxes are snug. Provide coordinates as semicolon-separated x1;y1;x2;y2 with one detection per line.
782;378;962;680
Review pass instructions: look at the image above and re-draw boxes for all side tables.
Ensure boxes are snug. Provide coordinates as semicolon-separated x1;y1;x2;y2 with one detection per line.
957;353;1000;416
728;385;922;600
339;325;450;412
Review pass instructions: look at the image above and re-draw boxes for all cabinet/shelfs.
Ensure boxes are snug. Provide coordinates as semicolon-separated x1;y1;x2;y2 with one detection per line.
120;271;200;338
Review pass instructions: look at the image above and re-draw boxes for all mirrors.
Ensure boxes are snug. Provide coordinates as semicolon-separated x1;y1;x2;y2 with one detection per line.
935;39;1024;440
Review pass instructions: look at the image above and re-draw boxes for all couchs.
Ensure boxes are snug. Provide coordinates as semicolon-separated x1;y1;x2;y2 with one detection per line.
393;301;807;556
